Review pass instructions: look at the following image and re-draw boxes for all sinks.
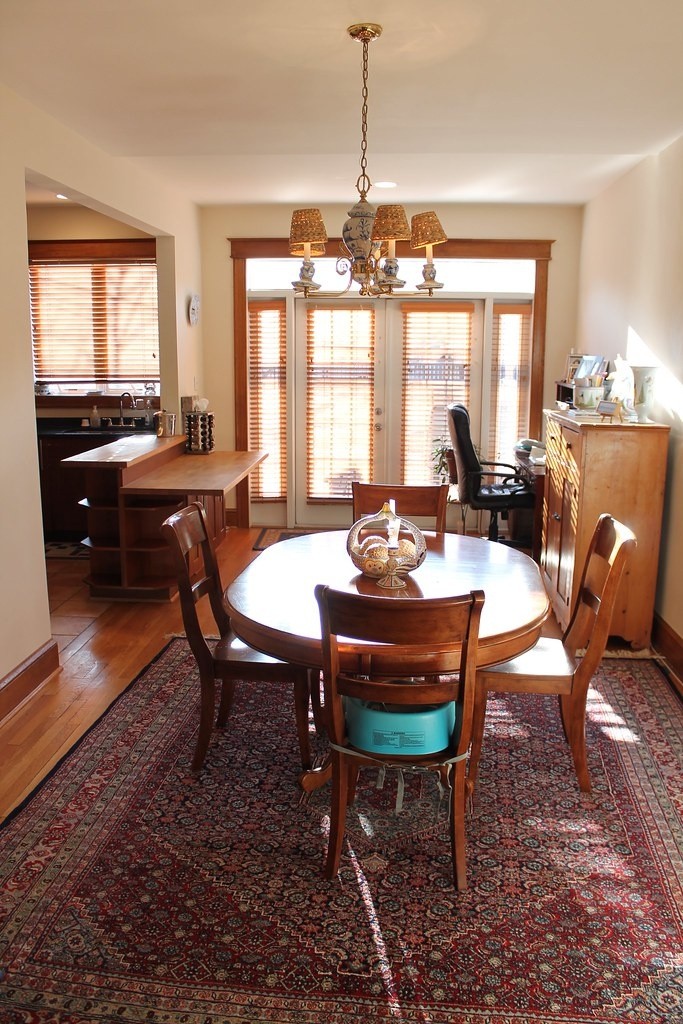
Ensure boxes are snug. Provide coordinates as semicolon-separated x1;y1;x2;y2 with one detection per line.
55;430;152;435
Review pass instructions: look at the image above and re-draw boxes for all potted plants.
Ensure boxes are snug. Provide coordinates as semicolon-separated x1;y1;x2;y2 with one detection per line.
430;437;486;484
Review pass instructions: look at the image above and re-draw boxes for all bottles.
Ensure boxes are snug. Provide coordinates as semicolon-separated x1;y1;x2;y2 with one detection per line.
90;405;100;428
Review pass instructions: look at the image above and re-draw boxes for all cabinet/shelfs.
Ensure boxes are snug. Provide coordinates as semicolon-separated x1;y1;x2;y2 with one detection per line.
541;408;672;651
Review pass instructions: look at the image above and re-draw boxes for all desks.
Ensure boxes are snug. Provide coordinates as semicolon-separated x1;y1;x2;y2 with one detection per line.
61;435;270;603
507;453;546;563
222;530;551;808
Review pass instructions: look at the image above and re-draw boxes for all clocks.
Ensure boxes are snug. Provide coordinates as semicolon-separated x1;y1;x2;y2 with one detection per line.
188;293;201;326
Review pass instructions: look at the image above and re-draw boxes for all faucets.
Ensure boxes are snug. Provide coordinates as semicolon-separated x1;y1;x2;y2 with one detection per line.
119;392;136;425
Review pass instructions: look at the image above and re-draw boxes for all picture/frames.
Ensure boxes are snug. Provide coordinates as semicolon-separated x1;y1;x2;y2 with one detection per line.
566;354;610;385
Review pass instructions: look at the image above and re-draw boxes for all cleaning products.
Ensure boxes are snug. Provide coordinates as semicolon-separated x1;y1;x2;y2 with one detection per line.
144;399;153;426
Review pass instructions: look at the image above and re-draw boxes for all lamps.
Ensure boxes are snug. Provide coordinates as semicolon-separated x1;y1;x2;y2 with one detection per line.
288;23;448;300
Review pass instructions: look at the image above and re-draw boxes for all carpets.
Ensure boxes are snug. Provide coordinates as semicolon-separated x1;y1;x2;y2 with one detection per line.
251;528;327;551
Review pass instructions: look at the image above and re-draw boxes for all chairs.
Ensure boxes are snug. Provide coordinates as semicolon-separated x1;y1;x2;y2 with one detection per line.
351;481;451;533
0;631;683;1024
448;402;535;550
470;512;637;795
159;502;323;773
314;584;485;890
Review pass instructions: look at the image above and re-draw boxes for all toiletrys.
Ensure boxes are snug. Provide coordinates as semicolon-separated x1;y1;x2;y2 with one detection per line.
90;405;101;428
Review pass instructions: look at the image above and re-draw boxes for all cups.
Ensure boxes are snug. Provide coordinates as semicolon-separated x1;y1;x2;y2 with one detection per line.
157;413;176;437
